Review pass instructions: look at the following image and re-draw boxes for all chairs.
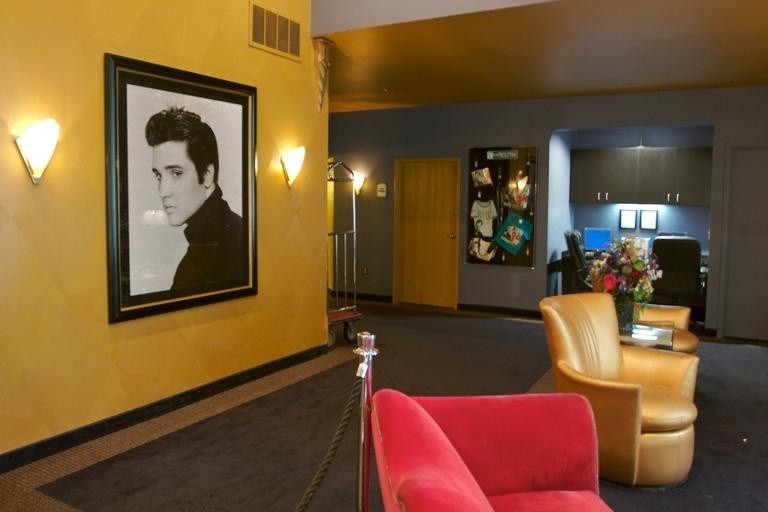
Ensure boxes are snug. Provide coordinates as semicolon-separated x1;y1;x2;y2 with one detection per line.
653;231;700;327
564;231;593;292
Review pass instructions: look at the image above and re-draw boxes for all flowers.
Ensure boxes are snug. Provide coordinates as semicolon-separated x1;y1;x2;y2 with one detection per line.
585;234;663;329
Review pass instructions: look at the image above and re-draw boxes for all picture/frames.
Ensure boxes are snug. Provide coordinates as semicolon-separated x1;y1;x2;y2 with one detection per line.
102;52;260;326
619;208;660;231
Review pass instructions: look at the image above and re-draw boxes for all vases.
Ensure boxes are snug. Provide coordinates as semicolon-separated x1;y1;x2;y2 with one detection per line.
612;291;635;337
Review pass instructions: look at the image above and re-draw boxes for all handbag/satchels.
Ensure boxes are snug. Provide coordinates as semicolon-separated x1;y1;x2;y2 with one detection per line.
468;237;497;262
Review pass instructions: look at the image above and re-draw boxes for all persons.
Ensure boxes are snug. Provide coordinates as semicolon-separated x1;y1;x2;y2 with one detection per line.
145;106;244;292
474;219;484;237
